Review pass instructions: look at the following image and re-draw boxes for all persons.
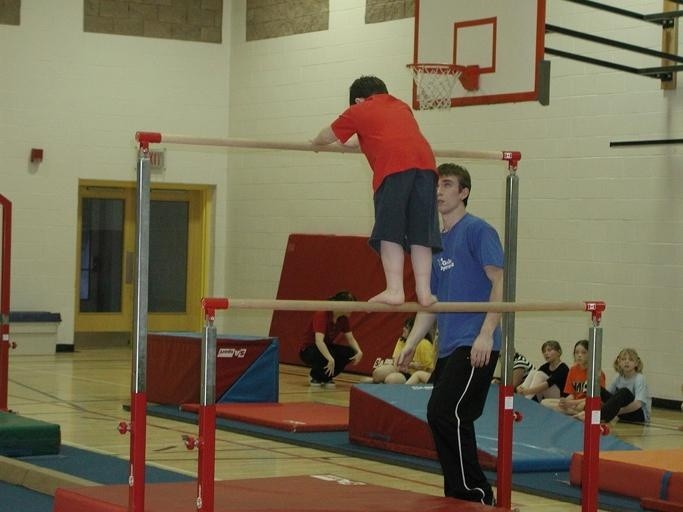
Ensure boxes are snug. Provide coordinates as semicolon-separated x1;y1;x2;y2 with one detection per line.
297;289;364;390
491;345;535;394
571;347;653;425
308;75;444;309
371;315;435;385
405;329;438;384
393;161;507;505
539;339;605;417
512;340;570;402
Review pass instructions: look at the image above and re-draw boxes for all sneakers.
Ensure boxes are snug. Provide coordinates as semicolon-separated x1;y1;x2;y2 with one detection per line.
308;369;337;387
